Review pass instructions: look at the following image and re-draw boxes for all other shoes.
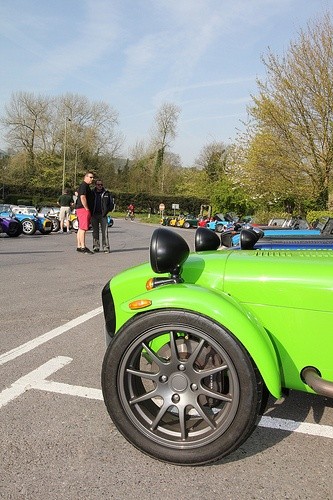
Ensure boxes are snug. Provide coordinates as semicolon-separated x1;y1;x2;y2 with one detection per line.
93;248;99;252
76;247;93;254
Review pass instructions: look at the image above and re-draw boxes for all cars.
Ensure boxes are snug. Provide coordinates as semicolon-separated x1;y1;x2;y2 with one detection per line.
160;214;333;250
97;228;333;467
0;204;114;238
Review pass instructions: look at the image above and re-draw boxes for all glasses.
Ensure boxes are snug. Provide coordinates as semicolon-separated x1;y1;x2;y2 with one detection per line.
89;177;94;179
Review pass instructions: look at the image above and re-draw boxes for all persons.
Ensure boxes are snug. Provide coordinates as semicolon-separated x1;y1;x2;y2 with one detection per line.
91;179;113;253
127;204;134;218
57;188;74;234
75;172;95;254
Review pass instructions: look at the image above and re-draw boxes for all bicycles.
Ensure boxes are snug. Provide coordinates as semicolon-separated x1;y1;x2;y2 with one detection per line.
125;209;136;221
